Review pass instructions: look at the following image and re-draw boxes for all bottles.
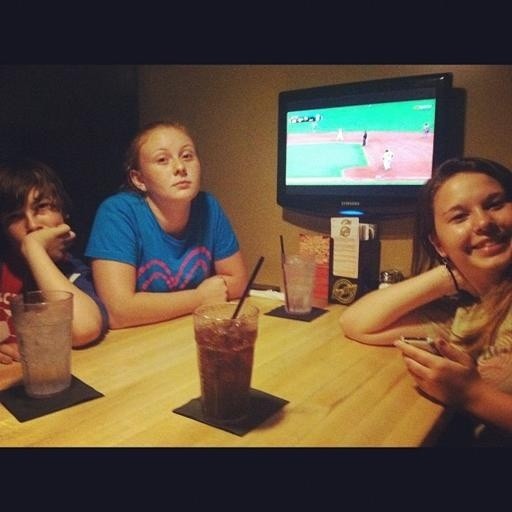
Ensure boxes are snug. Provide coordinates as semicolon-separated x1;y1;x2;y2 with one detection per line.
378;269;403;289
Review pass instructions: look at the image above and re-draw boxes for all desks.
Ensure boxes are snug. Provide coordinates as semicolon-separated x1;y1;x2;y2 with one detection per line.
0;285;450;448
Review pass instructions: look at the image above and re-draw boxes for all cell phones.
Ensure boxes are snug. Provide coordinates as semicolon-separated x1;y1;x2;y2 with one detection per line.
404;336;443;357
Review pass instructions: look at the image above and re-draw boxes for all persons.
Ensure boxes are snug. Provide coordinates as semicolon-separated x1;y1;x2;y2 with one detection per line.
336;127;345;142
382;149;394;170
339;158;512;434
423;124;431;136
0;159;108;365
362;130;367;146
84;121;249;330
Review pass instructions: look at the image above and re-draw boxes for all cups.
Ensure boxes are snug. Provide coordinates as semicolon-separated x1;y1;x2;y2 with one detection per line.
281;255;315;317
193;304;259;423
9;289;74;401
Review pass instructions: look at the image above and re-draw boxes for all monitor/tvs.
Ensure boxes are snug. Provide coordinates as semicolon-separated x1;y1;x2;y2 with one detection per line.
276;72;453;220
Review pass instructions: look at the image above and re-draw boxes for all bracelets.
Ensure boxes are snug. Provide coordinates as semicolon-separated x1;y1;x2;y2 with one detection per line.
446;262;461;297
